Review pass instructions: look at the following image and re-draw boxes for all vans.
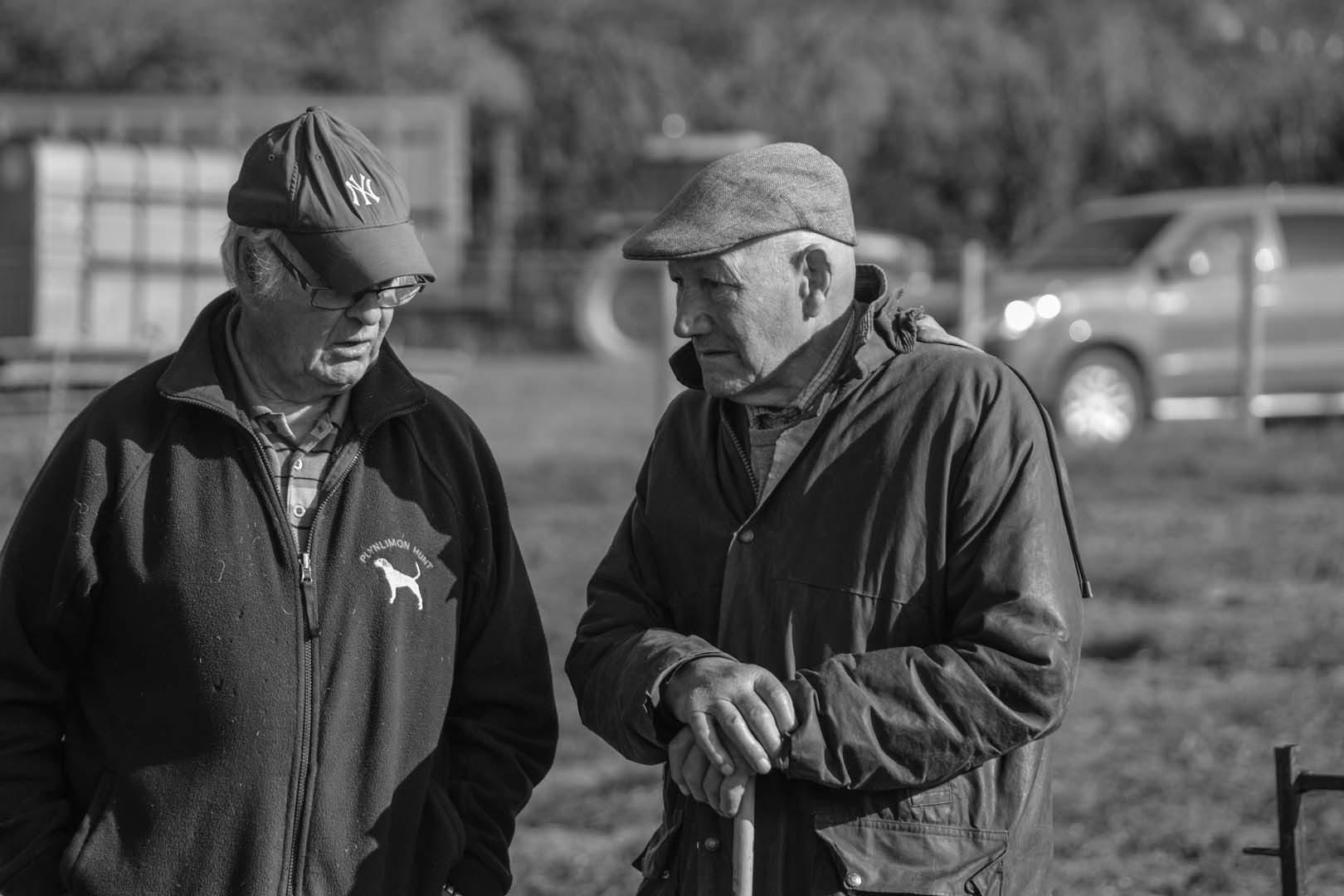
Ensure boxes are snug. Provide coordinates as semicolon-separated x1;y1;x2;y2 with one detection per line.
918;183;1343;449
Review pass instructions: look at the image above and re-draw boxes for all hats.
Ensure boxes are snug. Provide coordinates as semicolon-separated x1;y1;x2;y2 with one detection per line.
623;141;858;261
228;106;435;293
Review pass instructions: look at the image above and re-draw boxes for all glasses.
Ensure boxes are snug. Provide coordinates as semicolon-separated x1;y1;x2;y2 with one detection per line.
260;236;427;311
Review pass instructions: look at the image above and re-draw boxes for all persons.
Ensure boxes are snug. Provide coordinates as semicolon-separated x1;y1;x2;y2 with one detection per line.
564;142;1092;896
0;106;558;896
